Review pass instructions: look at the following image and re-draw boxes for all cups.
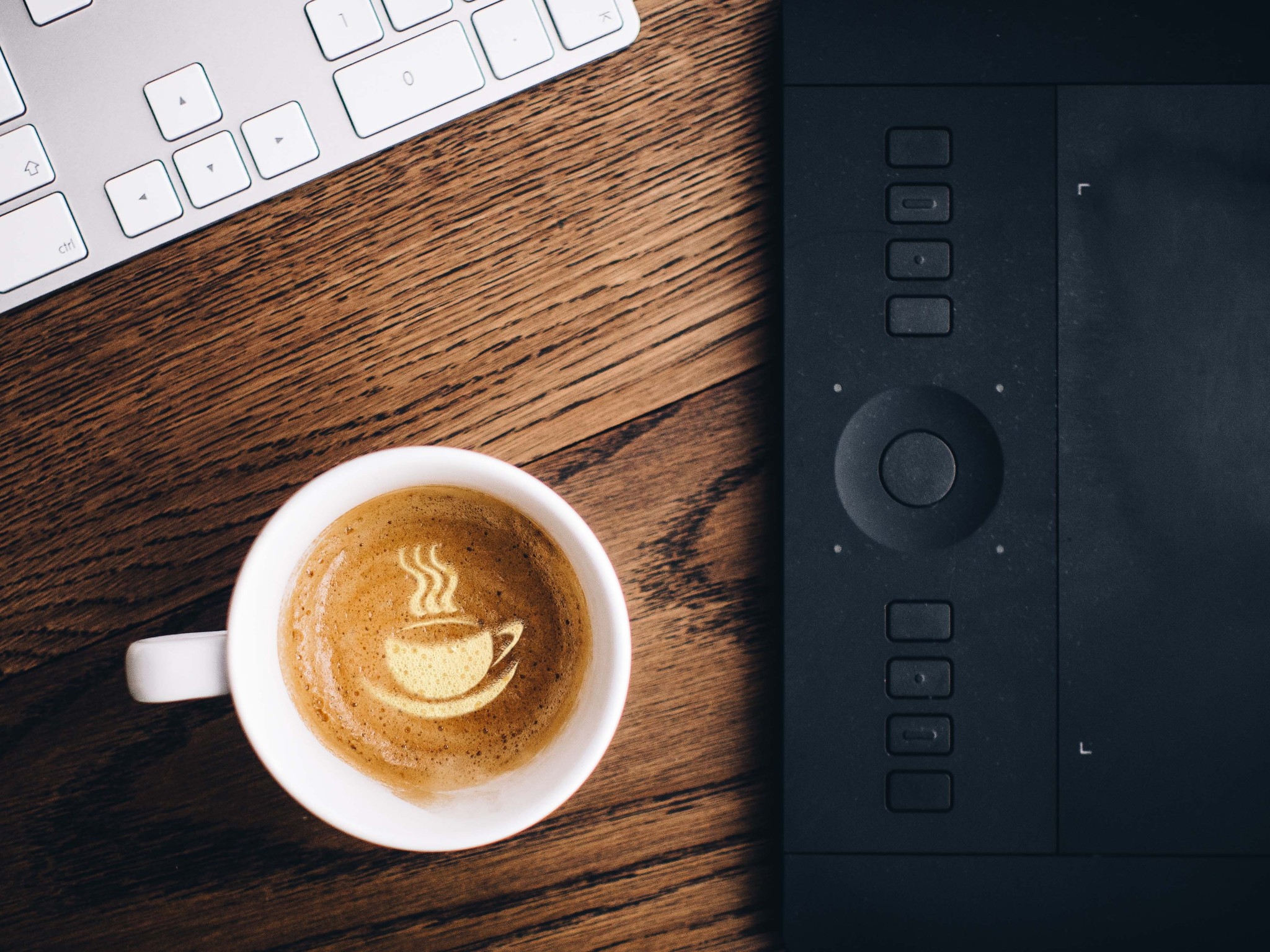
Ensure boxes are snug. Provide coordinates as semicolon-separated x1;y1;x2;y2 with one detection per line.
122;444;634;853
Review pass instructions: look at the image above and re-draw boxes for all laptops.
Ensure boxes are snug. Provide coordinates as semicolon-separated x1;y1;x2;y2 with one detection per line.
774;0;1270;952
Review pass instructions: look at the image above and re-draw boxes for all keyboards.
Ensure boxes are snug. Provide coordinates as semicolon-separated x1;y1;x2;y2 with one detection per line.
0;0;643;311
